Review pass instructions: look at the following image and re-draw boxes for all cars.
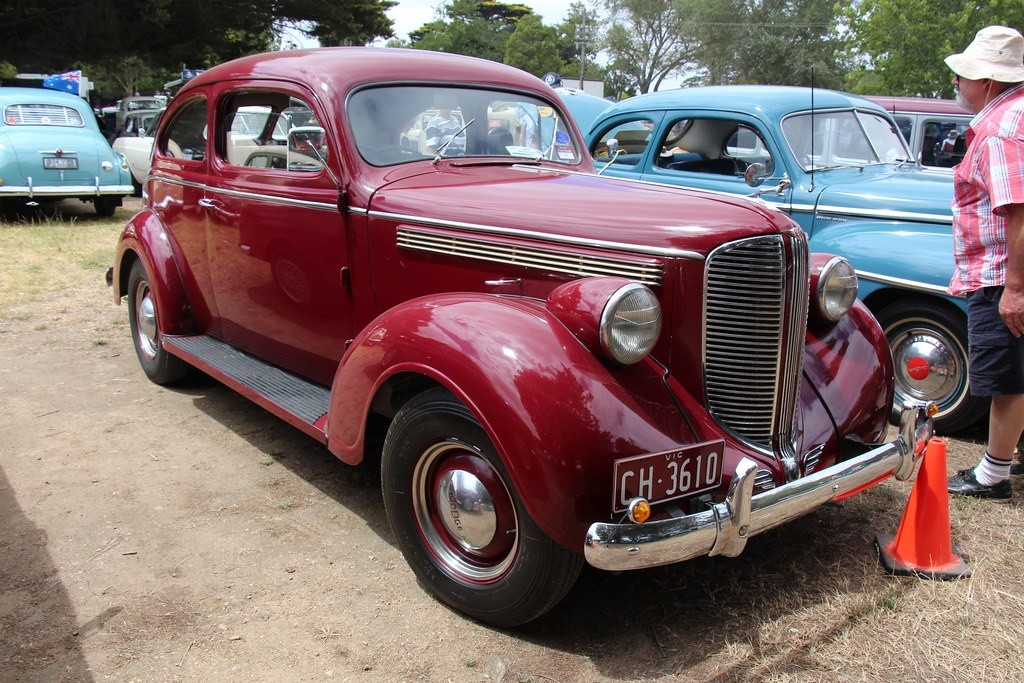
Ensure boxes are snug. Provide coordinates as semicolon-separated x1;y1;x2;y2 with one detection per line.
107;46;939;627
0;86;991;436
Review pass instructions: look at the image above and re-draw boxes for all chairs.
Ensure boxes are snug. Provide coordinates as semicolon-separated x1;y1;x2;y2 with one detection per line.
667;159;737;176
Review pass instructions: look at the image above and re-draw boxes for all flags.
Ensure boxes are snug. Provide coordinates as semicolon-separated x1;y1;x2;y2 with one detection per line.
43;70;82;94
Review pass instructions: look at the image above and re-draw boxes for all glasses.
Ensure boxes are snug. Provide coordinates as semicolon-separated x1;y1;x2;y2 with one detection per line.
956;74;961;82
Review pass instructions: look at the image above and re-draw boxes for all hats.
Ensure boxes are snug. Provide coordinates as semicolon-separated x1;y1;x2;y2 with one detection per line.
943;25;1024;82
947;130;960;138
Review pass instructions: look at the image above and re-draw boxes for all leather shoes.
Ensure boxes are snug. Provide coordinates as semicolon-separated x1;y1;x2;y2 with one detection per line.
946;468;1014;502
959;450;1024;479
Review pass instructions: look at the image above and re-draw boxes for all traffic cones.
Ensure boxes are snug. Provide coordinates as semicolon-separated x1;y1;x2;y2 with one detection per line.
873;438;974;579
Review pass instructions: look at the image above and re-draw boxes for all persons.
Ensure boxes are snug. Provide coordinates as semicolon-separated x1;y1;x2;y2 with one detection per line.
421;72;566;155
303;112;329;147
135;90;175;106
932;130;960;156
944;25;1024;504
